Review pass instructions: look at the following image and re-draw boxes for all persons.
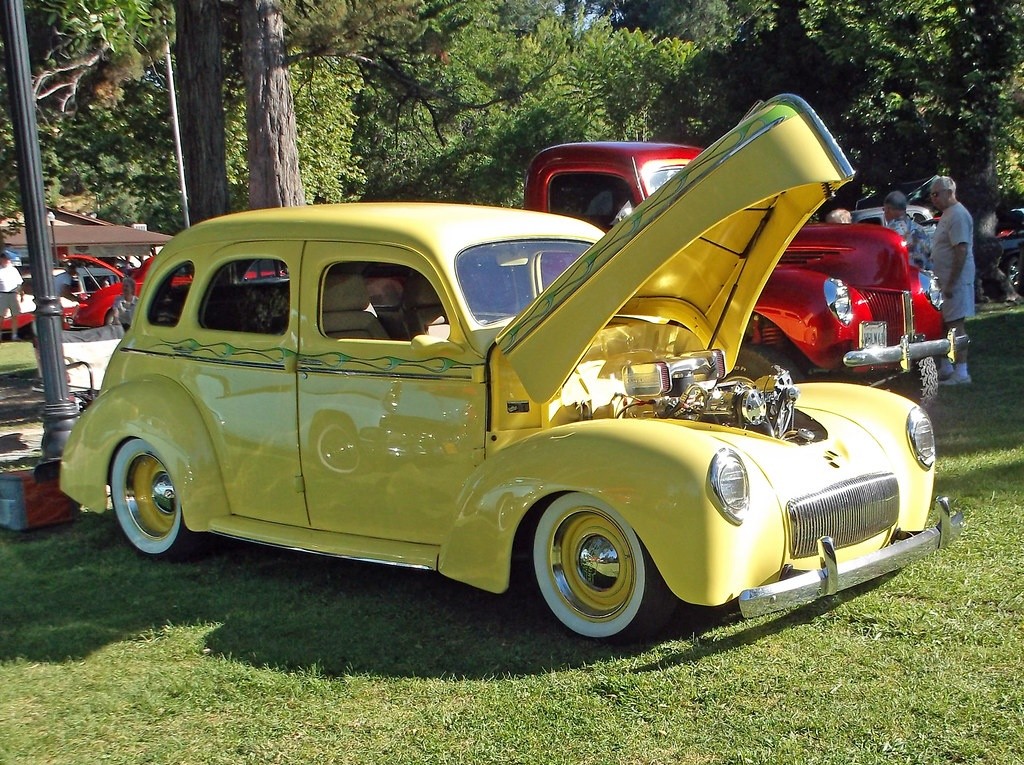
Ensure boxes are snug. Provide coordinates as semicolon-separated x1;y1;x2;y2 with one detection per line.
113;277;139;333
826;209;851;223
101;276;109;288
882;191;931;270
0;251;25;343
53;263;88;330
929;176;976;386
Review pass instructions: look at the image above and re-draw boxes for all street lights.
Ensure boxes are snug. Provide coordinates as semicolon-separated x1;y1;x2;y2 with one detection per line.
47;211;59;268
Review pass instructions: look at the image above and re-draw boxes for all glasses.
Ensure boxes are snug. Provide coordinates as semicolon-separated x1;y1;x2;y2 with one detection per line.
929;188;948;199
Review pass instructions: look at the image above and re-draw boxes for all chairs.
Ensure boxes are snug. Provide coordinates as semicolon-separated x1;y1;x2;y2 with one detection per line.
323;274;390;340
403;274;450;341
61;322;122;404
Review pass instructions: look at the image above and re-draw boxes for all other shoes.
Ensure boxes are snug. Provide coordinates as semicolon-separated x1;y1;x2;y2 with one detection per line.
938;368;952;381
939;373;972;387
11;335;22;342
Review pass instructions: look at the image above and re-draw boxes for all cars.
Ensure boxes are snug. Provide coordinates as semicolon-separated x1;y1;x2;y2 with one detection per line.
517;139;972;414
1;254;293;342
848;174;1024;299
56;91;964;648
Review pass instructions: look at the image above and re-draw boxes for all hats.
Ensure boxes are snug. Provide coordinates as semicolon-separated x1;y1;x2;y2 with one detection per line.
0;253;12;261
